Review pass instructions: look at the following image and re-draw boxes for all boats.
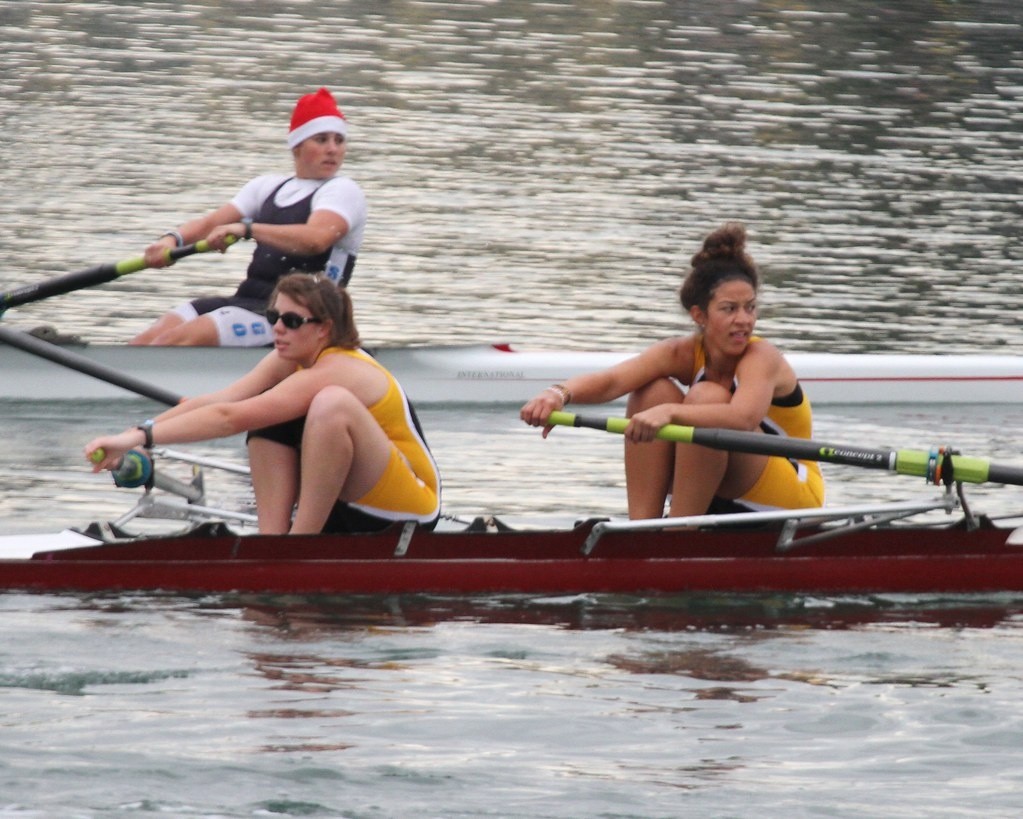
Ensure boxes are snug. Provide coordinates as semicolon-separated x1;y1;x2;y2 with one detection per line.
0;333;1023;405
0;520;1023;593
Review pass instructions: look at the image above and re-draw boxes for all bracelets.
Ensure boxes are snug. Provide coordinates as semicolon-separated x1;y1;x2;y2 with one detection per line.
138;425;153;448
546;383;571;405
161;231;183;247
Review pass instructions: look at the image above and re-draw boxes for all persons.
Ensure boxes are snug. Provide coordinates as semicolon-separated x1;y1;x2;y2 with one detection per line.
84;272;441;537
130;87;368;346
521;225;827;520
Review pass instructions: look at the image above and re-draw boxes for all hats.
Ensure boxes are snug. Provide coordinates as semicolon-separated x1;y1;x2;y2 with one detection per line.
287;87;347;148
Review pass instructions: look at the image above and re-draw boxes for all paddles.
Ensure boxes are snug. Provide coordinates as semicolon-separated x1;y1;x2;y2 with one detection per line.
0;234;239;313
89;446;207;505
531;408;1023;487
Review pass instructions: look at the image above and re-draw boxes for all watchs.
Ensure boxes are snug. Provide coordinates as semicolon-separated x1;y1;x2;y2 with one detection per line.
241;219;253;239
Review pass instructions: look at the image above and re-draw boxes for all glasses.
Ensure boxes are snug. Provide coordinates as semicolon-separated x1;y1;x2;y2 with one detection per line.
265;309;321;329
